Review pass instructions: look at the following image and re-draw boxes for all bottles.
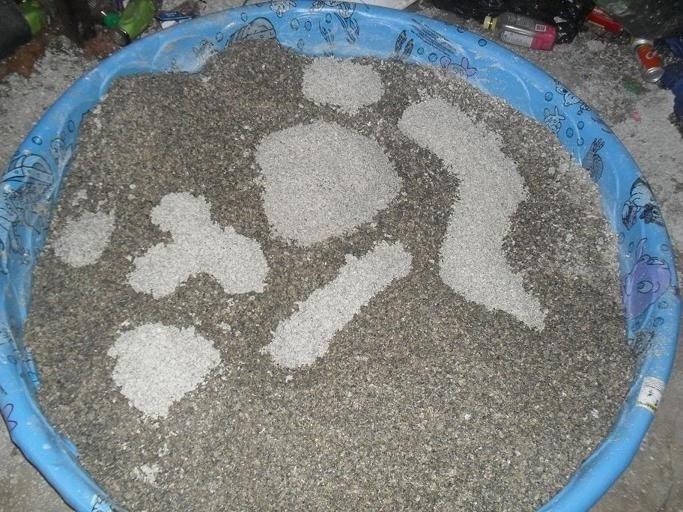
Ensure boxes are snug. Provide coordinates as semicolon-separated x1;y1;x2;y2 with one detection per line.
484;11;557;52
87;0;162;50
